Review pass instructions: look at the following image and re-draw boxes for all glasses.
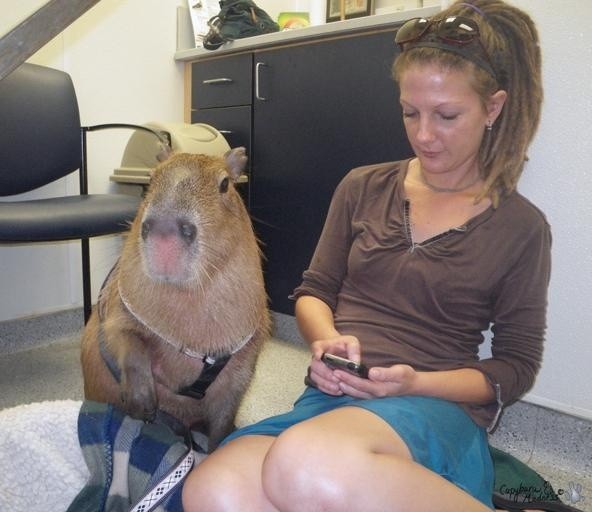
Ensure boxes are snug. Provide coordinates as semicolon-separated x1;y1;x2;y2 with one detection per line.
395;16;497;74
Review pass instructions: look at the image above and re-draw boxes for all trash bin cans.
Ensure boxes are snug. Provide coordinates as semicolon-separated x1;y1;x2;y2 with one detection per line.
110;123;248;196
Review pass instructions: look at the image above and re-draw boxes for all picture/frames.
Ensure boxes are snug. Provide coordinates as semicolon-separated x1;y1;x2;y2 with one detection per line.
322;0;376;23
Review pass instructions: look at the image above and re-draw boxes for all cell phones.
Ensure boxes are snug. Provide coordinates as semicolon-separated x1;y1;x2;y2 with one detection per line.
320;351;367;380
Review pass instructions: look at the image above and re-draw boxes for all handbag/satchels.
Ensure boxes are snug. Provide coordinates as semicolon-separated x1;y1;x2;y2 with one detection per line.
202;1;280;50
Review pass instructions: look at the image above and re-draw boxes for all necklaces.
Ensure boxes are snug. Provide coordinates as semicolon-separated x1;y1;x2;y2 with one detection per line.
418;166;482;193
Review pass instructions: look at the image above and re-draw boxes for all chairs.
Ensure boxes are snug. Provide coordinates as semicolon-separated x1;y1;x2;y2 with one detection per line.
0;60;174;341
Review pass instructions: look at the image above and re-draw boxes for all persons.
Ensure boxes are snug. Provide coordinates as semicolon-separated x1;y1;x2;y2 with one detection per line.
179;0;553;511
